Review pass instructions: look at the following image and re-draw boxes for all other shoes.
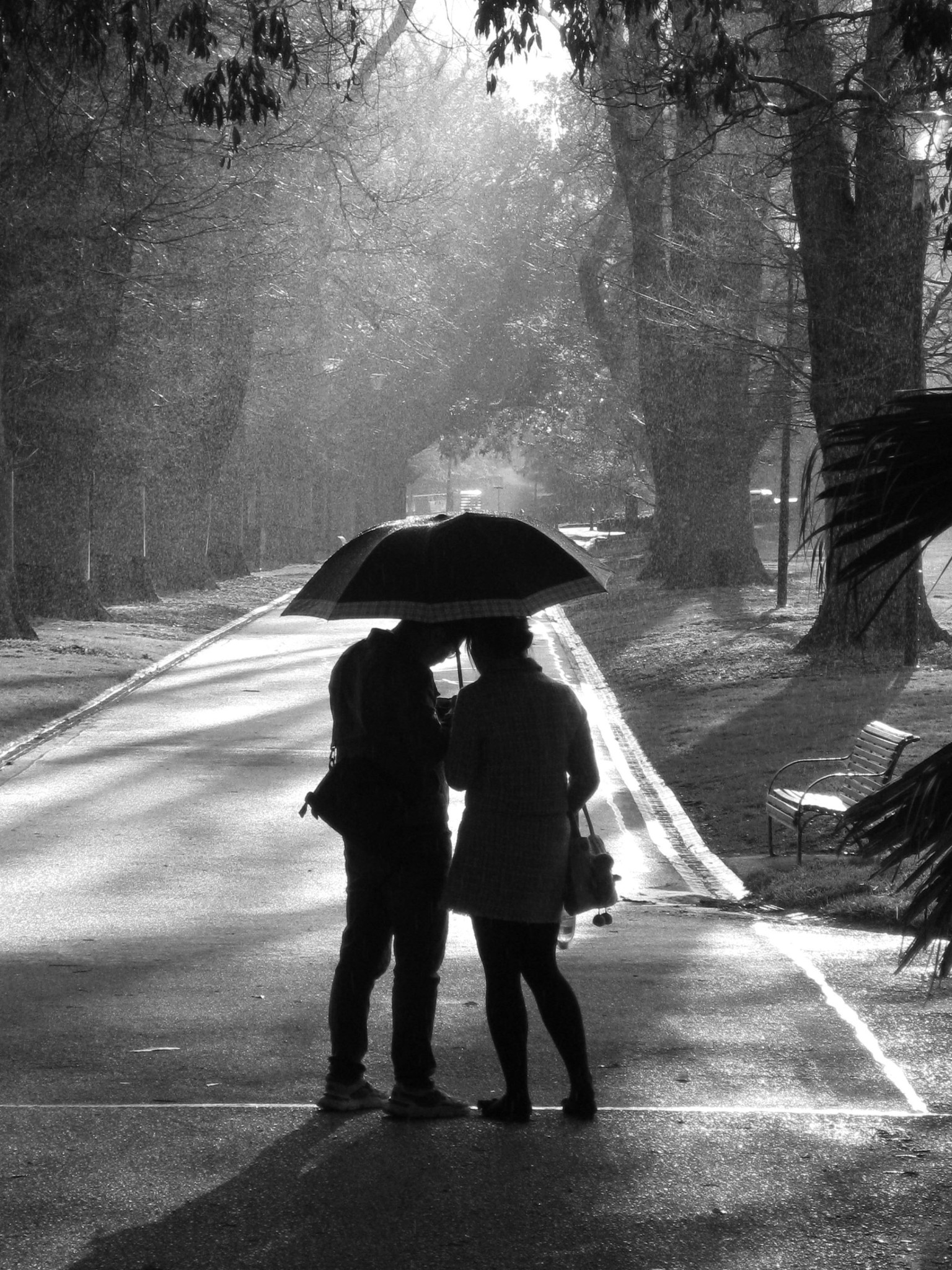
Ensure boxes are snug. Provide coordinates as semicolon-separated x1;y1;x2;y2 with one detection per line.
317;1072;391;1111
560;1094;598;1120
383;1078;471;1121
475;1097;534;1121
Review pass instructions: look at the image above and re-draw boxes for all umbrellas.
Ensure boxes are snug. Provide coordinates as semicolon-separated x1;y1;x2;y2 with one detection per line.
281;510;616;690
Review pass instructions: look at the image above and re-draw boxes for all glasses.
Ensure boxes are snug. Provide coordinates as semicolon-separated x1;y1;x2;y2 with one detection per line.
442;633;461;659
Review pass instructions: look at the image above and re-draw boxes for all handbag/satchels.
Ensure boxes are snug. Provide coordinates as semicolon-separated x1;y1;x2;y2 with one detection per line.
298;742;362;838
563;801;622;927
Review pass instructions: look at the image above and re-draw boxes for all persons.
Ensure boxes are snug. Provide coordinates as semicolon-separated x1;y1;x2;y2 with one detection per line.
446;619;601;1124
304;621;469;1121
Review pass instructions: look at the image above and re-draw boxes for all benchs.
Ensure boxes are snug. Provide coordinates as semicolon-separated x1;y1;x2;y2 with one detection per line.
765;720;921;867
